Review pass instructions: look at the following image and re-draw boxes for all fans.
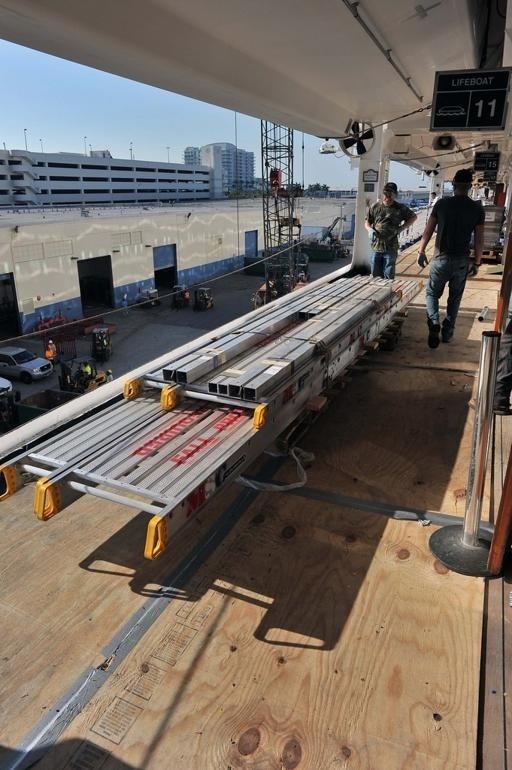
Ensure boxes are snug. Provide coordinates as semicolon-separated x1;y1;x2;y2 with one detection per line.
340;103;434;157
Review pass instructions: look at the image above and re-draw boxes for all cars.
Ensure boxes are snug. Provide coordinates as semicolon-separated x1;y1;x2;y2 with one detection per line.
0;377;13;397
0;346;55;384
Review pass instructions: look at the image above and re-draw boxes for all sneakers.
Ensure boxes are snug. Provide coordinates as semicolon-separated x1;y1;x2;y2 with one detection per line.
443;336;453;342
428;320;440;348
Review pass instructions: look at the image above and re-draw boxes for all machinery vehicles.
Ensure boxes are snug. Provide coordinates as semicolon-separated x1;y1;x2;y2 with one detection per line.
300;216;351;263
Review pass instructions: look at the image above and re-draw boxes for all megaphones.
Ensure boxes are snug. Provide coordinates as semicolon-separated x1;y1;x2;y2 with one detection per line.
432;133;456;150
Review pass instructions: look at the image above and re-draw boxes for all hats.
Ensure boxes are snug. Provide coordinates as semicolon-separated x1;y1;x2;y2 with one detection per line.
384;182;397;193
455;169;473;183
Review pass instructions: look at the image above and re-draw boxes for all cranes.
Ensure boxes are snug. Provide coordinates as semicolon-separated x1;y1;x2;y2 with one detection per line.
255;120;310;309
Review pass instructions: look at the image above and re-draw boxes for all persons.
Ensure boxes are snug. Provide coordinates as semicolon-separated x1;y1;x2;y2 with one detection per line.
103;369;113;385
200;291;208;303
46;346;55;372
430;191;440;207
98;329;108;366
122;298;129;317
82;360;92;376
418;170;484;350
183;287;190;306
364;182;417;280
48;340;56;353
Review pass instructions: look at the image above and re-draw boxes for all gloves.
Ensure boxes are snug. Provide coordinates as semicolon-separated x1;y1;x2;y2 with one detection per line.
417;250;428;268
368;229;377;241
468;263;479;277
